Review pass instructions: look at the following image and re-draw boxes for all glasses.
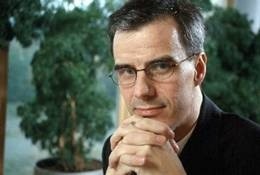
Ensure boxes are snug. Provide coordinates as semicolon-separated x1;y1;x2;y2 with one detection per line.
108;52;199;86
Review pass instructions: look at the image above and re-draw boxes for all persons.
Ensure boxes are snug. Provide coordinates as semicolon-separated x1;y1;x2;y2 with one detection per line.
102;0;260;175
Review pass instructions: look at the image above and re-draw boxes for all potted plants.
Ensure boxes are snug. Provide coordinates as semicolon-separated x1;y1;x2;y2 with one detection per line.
8;1;115;175
